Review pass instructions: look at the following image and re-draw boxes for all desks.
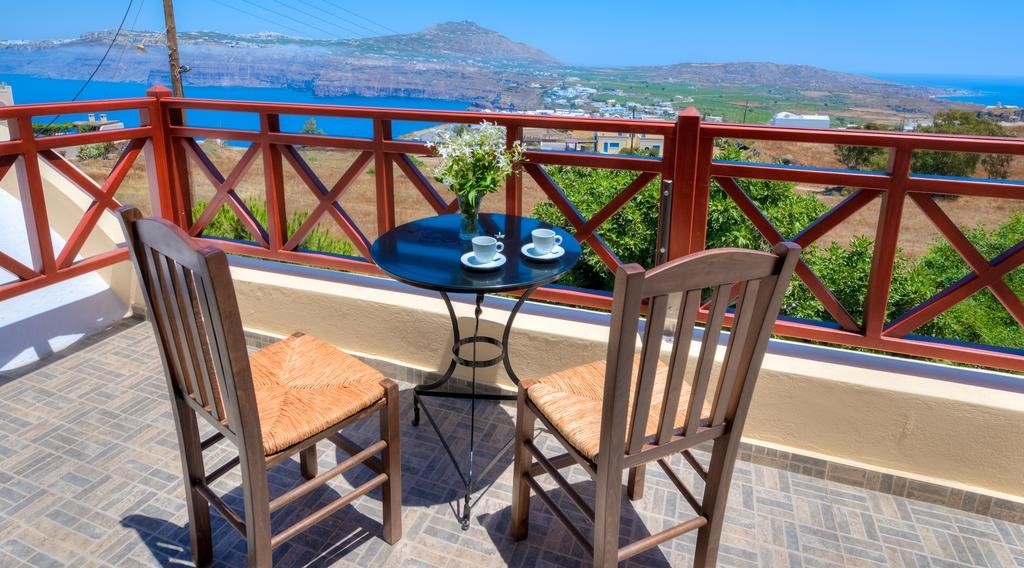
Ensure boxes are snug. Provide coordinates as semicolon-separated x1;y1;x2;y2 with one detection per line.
370;213;582;530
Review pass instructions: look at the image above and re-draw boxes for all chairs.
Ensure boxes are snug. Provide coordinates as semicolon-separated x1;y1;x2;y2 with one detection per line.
506;242;802;568
117;203;402;568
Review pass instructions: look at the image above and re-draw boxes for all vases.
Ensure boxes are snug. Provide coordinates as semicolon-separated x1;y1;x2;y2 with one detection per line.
458;194;483;240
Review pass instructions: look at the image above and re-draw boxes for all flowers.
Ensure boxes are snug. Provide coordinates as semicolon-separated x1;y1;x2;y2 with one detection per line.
423;120;530;200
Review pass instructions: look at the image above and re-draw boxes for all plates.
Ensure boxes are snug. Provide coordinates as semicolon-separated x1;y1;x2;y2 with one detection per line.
521;243;565;259
461;251;507;269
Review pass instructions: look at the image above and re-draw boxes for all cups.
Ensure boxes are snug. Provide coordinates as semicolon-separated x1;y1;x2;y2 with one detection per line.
472;236;504;264
531;228;563;254
